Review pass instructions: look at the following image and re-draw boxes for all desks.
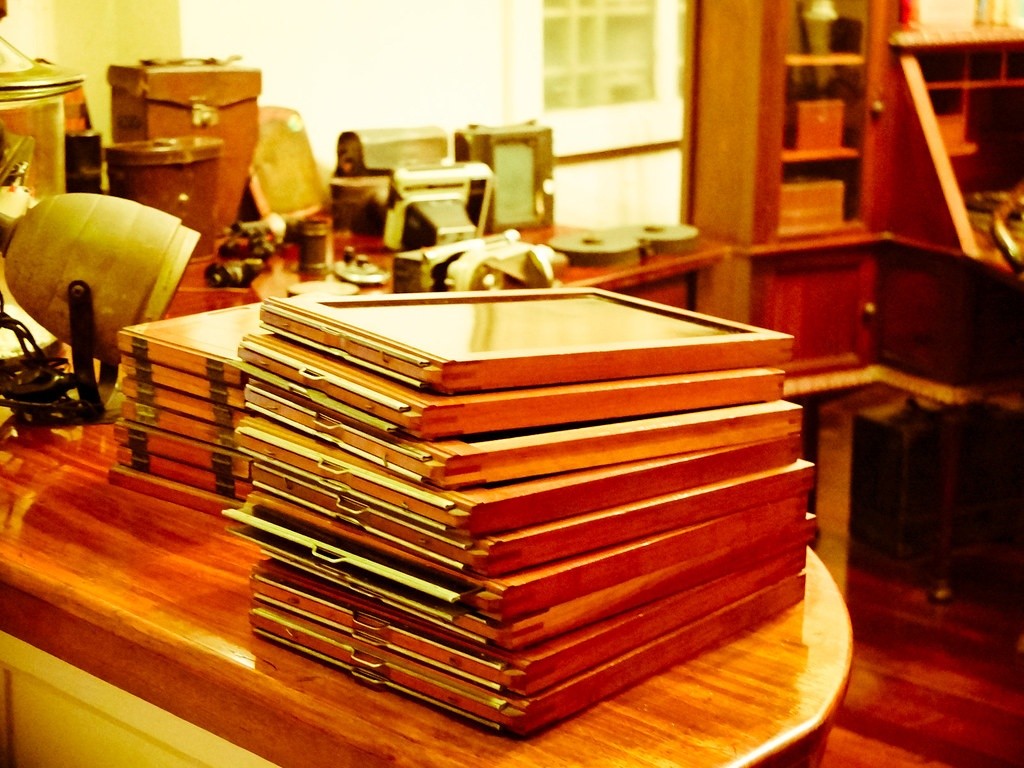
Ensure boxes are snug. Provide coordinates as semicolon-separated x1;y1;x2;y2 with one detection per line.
0;230;854;767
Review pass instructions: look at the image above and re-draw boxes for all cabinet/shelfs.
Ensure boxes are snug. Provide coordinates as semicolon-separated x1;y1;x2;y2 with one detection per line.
684;1;1024;606
